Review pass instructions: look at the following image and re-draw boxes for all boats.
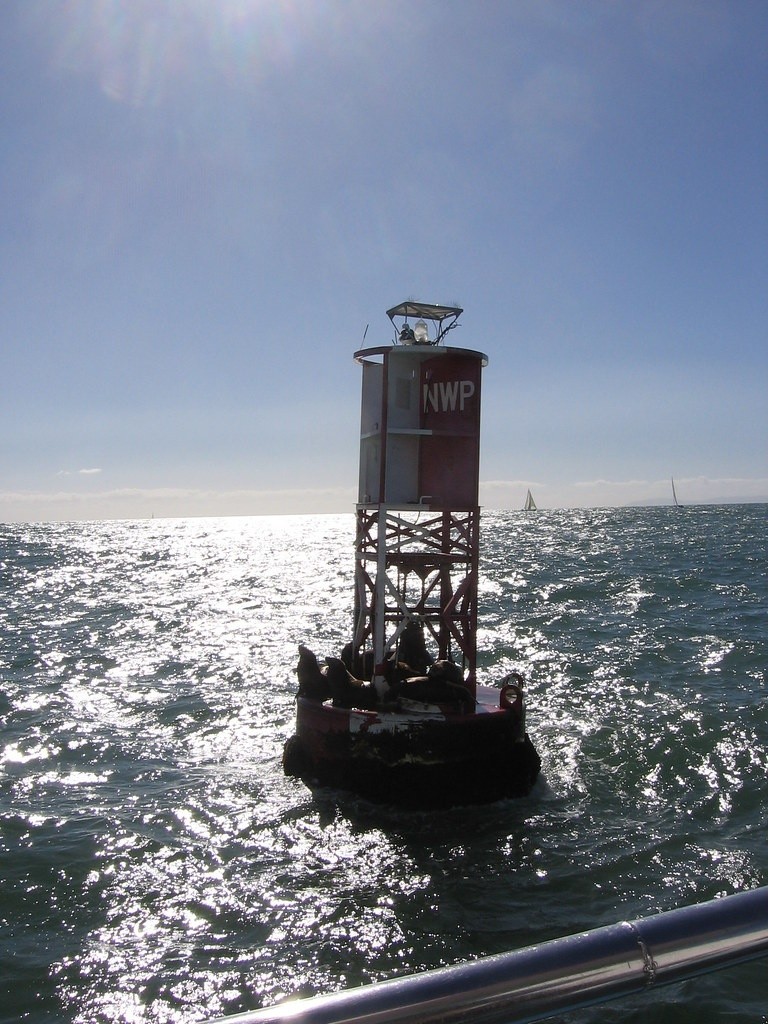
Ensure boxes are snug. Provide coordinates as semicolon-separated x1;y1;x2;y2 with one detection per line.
282;648;541;809
